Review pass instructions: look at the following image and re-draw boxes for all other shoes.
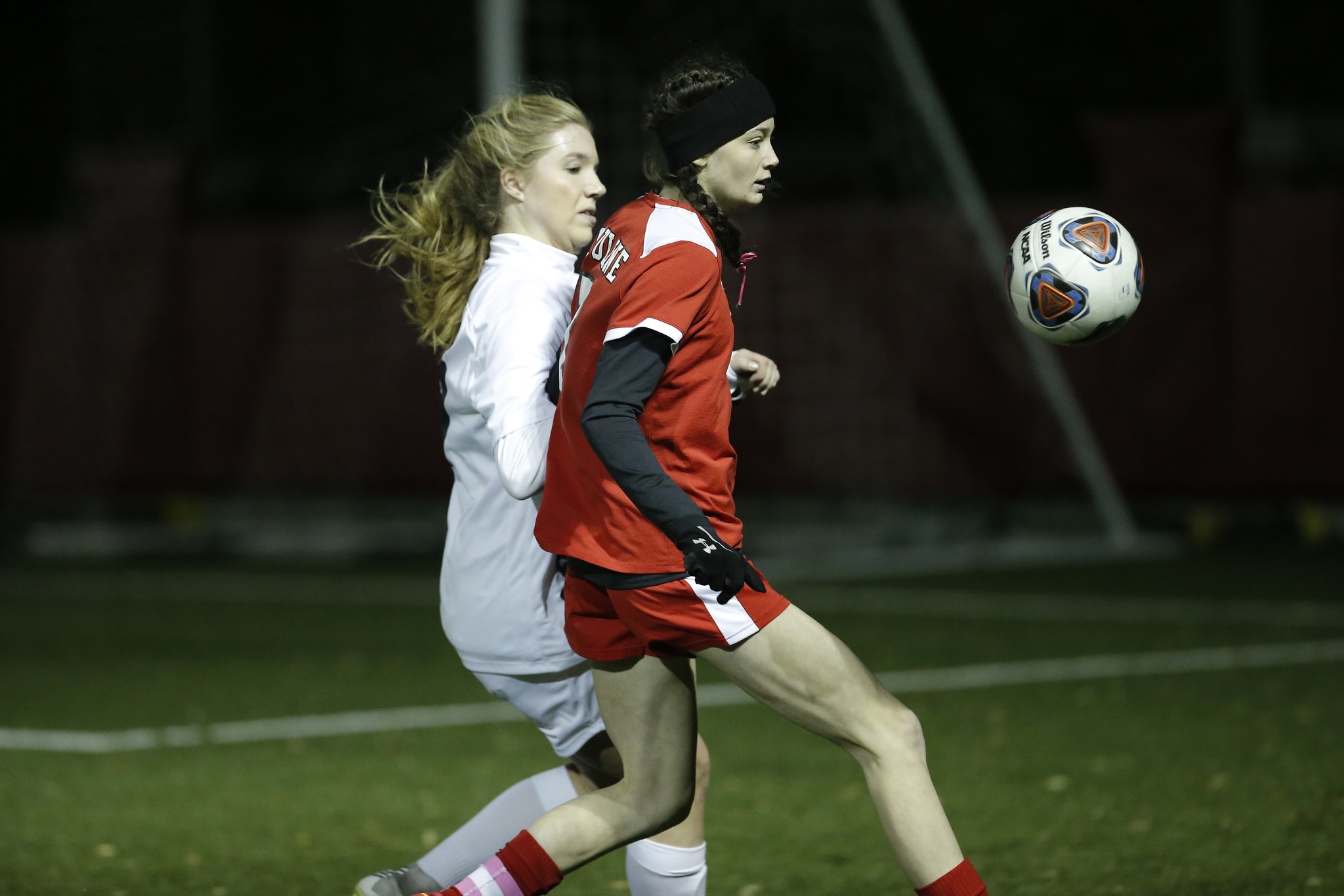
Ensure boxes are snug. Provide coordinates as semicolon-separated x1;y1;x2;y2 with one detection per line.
352;867;408;896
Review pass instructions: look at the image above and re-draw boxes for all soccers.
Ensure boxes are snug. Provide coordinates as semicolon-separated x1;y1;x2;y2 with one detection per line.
1003;206;1147;348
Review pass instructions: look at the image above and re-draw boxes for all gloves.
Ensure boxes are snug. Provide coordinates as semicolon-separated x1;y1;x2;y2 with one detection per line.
665;516;767;604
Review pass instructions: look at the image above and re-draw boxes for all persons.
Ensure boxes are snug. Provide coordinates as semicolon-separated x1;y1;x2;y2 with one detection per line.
353;94;781;896
416;68;986;896
